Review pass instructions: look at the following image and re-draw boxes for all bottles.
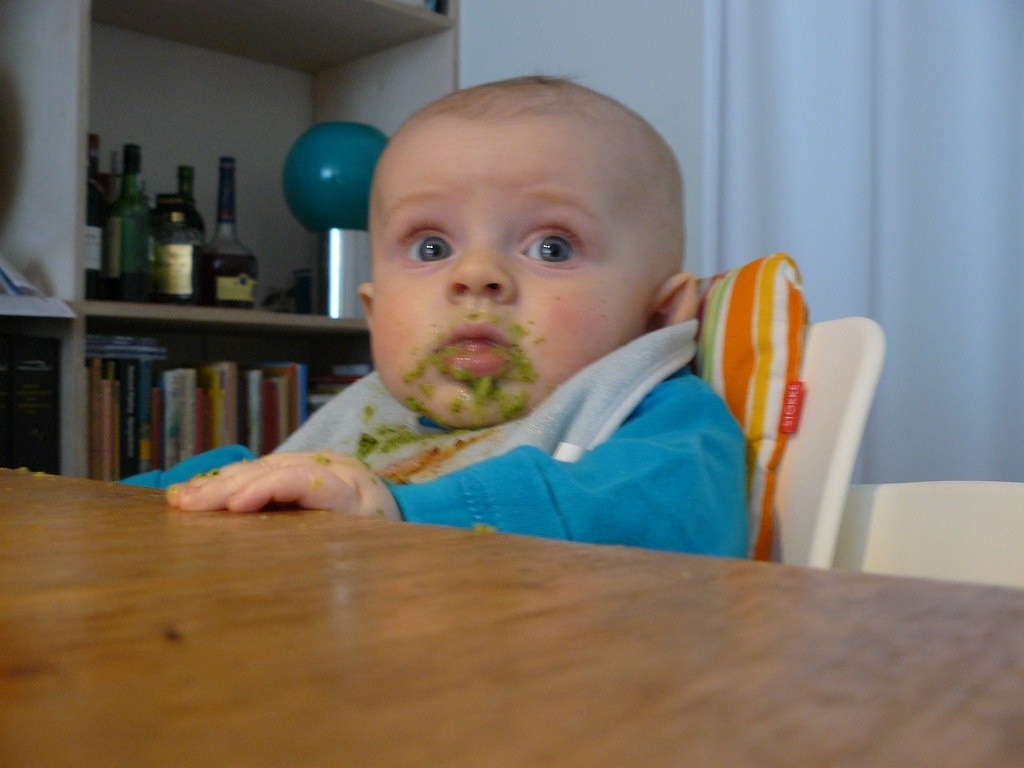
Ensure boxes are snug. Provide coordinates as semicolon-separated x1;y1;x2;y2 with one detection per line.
86;134;259;307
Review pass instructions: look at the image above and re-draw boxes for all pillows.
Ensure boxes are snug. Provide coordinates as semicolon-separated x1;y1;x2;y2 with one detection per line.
689;256;808;562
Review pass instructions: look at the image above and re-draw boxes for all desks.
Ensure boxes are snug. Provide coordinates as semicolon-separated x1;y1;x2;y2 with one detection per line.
0;468;1023;768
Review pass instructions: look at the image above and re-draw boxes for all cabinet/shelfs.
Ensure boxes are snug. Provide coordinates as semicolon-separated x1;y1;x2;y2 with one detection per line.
0;0;460;481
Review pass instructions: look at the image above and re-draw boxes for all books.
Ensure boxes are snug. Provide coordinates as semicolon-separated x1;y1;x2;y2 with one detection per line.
85;334;370;482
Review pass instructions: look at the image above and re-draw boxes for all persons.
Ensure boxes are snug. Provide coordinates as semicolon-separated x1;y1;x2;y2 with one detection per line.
117;75;746;561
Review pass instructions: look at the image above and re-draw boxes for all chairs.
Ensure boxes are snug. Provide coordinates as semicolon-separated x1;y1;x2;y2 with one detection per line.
831;479;1024;592
770;254;887;569
